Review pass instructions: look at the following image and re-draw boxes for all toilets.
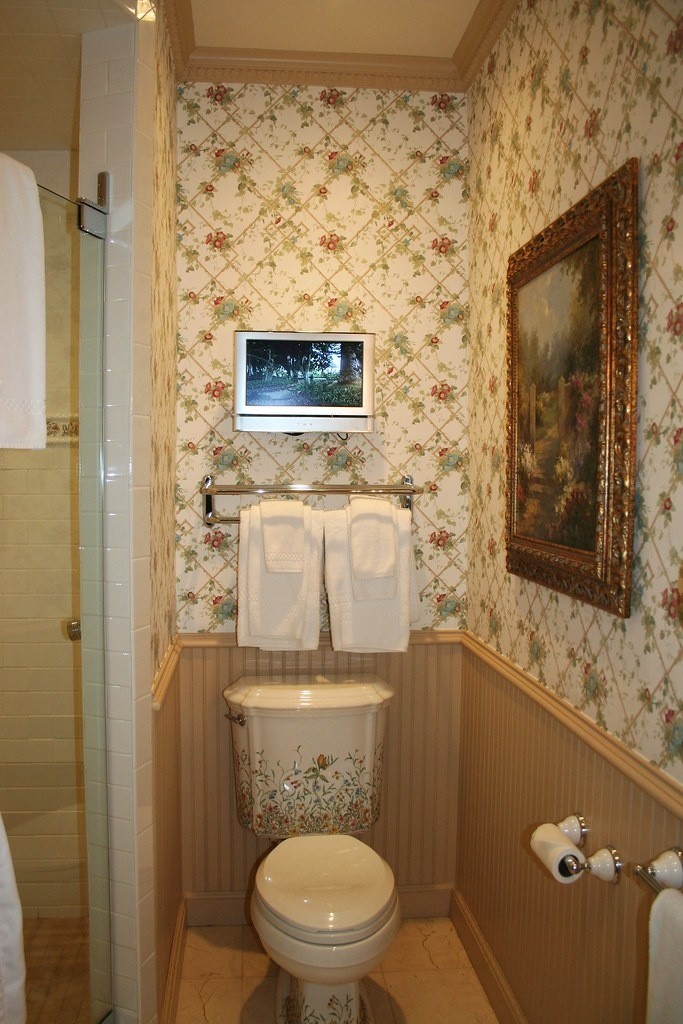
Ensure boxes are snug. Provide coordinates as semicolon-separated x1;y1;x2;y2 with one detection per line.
219;670;406;1024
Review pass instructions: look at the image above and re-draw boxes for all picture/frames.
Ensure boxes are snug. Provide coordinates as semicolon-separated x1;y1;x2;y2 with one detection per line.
503;155;638;619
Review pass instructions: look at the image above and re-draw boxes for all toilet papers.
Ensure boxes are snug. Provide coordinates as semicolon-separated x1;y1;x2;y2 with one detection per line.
530;819;588;887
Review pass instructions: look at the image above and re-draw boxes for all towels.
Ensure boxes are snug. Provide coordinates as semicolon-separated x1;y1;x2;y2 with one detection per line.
643;888;683;1024
0;150;46;451
232;494;418;654
